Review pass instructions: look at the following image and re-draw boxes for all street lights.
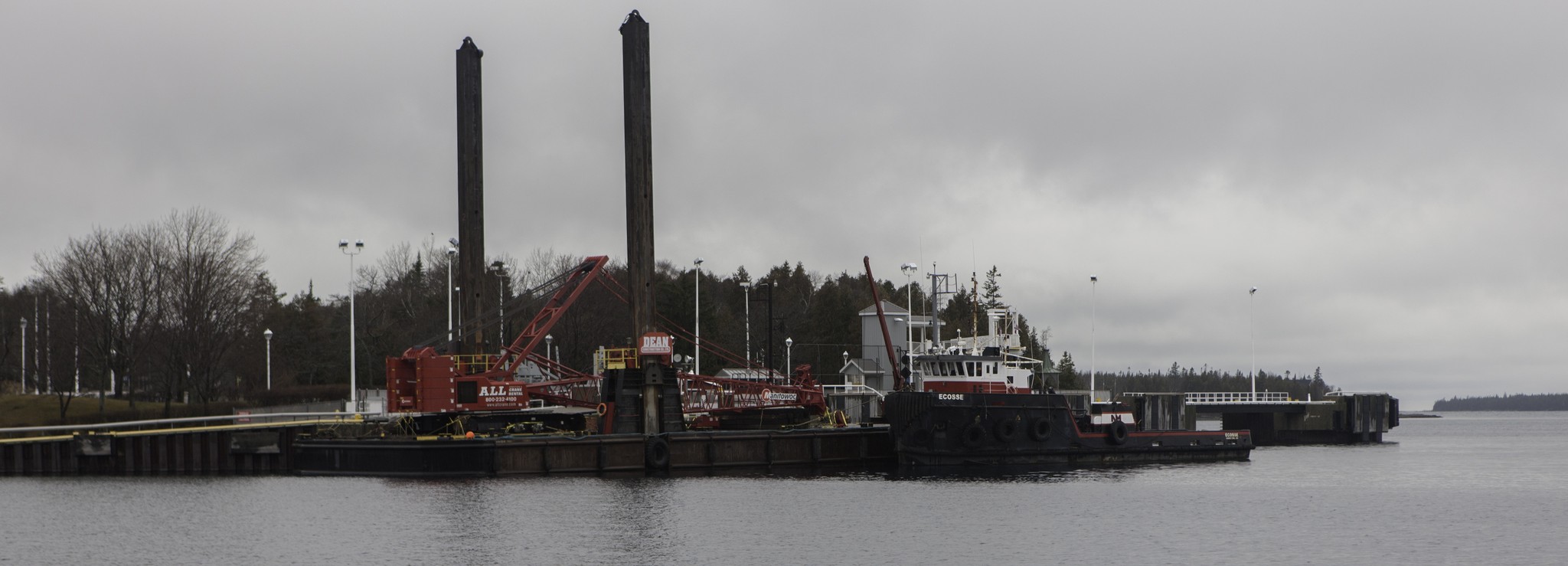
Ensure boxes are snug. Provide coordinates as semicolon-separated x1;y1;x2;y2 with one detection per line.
446;247;456;355
900;262;918;391
338;238;365;412
1089;273;1098;406
740;282;750;369
785;337;793;386
263;328;273;391
693;257;704;376
1249;286;1258;403
19;316;29;395
490;264;511;354
843;350;848;390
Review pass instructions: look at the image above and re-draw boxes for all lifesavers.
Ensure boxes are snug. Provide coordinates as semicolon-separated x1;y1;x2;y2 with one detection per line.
1032;417;1052;441
645;437;670;468
994;418;1017;442
960;423;987;450
1111;421;1128;445
914;428;932;445
597;403;606;416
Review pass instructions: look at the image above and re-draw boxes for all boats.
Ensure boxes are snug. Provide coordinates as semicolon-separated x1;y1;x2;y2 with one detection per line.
862;255;1258;470
